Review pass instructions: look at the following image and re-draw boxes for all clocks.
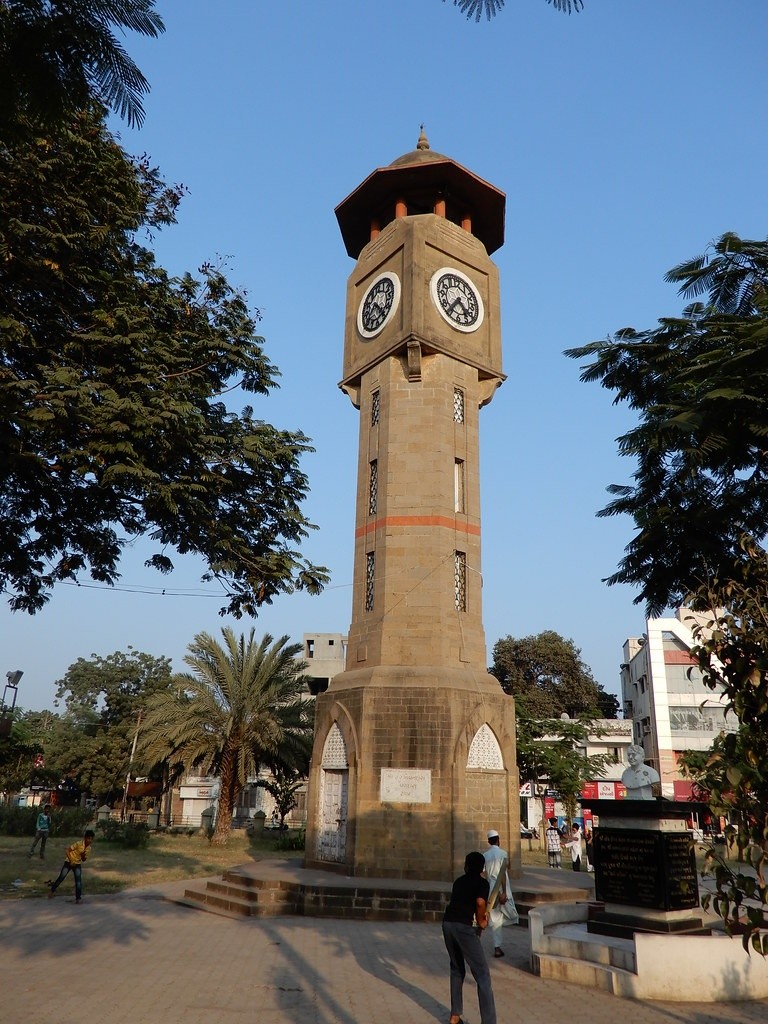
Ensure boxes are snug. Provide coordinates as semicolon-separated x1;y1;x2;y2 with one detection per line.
437;274;479;326
362;277;394;332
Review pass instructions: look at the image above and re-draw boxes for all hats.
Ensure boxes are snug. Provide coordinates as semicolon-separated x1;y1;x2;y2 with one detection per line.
487;830;498;838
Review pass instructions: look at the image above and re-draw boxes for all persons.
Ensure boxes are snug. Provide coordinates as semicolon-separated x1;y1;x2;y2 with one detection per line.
480;830;510;959
519;819;533;839
48;830;95;904
561;822;582;872
704;814;715;837
585;827;595;873
441;851;498;1024
621;745;661;799
29;805;51;861
546;818;578;870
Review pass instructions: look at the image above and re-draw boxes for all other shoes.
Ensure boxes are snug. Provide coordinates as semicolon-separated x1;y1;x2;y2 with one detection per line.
494;949;504;958
40;853;44;859
48;888;56;898
456;1017;464;1024
28;851;34;859
75;899;86;905
